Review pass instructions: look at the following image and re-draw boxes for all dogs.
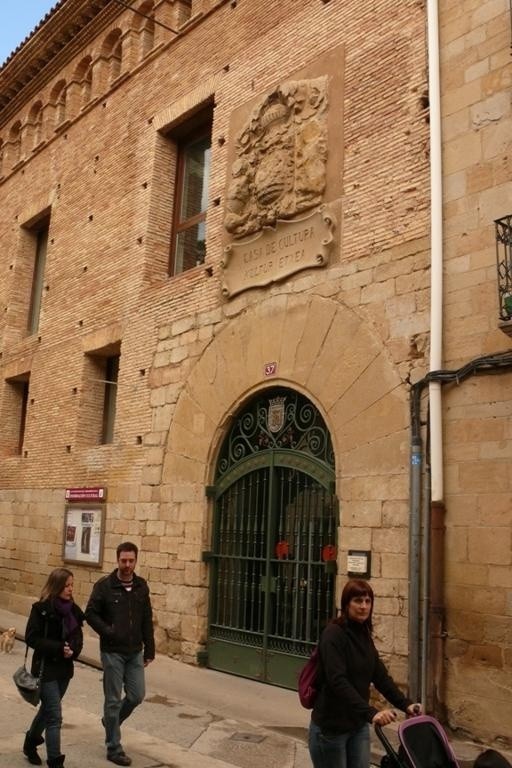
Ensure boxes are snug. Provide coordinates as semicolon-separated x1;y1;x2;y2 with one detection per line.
0;626;18;656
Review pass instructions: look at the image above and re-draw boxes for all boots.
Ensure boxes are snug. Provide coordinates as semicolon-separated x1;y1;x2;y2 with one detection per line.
23;729;44;765
47;755;65;768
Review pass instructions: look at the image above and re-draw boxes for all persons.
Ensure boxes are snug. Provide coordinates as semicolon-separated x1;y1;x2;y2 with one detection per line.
21;566;86;766
83;540;158;765
308;578;424;767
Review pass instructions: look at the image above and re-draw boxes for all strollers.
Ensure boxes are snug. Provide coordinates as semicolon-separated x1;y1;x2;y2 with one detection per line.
374;706;512;768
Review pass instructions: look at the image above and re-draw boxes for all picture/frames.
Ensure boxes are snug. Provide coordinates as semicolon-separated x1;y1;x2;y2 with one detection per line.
60;503;107;566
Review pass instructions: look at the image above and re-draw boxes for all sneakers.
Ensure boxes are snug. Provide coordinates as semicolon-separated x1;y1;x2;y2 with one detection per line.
102;717;106;727
107;752;131;766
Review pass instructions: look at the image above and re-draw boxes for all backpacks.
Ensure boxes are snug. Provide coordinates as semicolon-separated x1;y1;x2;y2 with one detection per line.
297;616;344;710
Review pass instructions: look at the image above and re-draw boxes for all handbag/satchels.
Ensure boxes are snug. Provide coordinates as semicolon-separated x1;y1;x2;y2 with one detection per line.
13;666;42;707
